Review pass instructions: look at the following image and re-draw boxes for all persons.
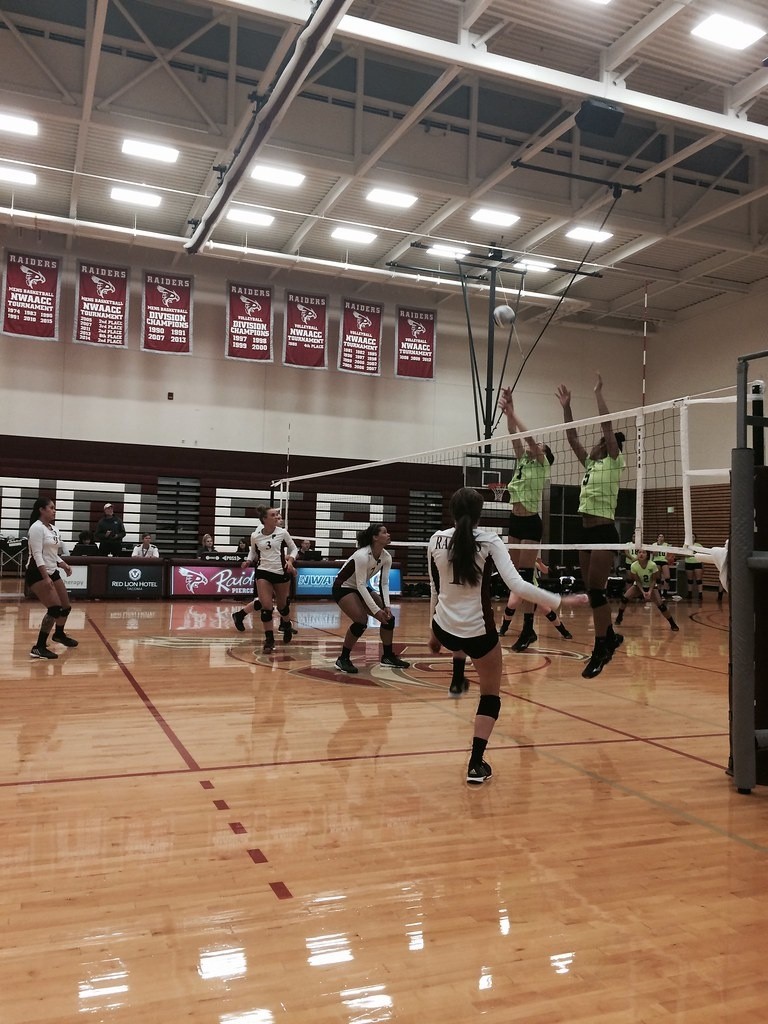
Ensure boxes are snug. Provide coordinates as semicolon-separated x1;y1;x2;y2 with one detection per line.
240;504;298;653
497;386;573;651
650;533;673;600
427;486;576;783
197;534;218;560
70;530;104;556
624;533;638;591
236;537;250;552
232;513;299;634
716;582;723;601
95;503;125;557
683;532;703;600
25;496;79;660
553;369;626;680
296;539;328;561
615;549;679;631
131;533;159;558
332;523;410;674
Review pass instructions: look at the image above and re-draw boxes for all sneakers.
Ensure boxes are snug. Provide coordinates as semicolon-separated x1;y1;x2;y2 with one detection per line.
582;634;623;679
467;759;492;783
52;633;78;647
31;646;58;660
448;679;469;697
512;630;537;651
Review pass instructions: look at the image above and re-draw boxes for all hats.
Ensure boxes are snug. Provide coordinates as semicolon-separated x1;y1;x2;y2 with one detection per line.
104;503;114;510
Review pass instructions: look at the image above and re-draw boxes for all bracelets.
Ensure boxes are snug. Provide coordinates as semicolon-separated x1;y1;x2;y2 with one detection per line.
150;556;151;558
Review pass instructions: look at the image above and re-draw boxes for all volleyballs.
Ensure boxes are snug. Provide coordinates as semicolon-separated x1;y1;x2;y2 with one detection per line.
492;304;516;328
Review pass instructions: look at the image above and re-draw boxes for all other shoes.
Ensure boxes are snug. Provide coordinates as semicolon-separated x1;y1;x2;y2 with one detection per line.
279;617;298;634
698;596;703;600
263;640;272;654
334;656;358;673
233;612;245;631
671;624;679;631
497;625;508;636
283;620;293;642
562;631;573;639
685;595;692;600
380;655;410;668
615;617;623;625
